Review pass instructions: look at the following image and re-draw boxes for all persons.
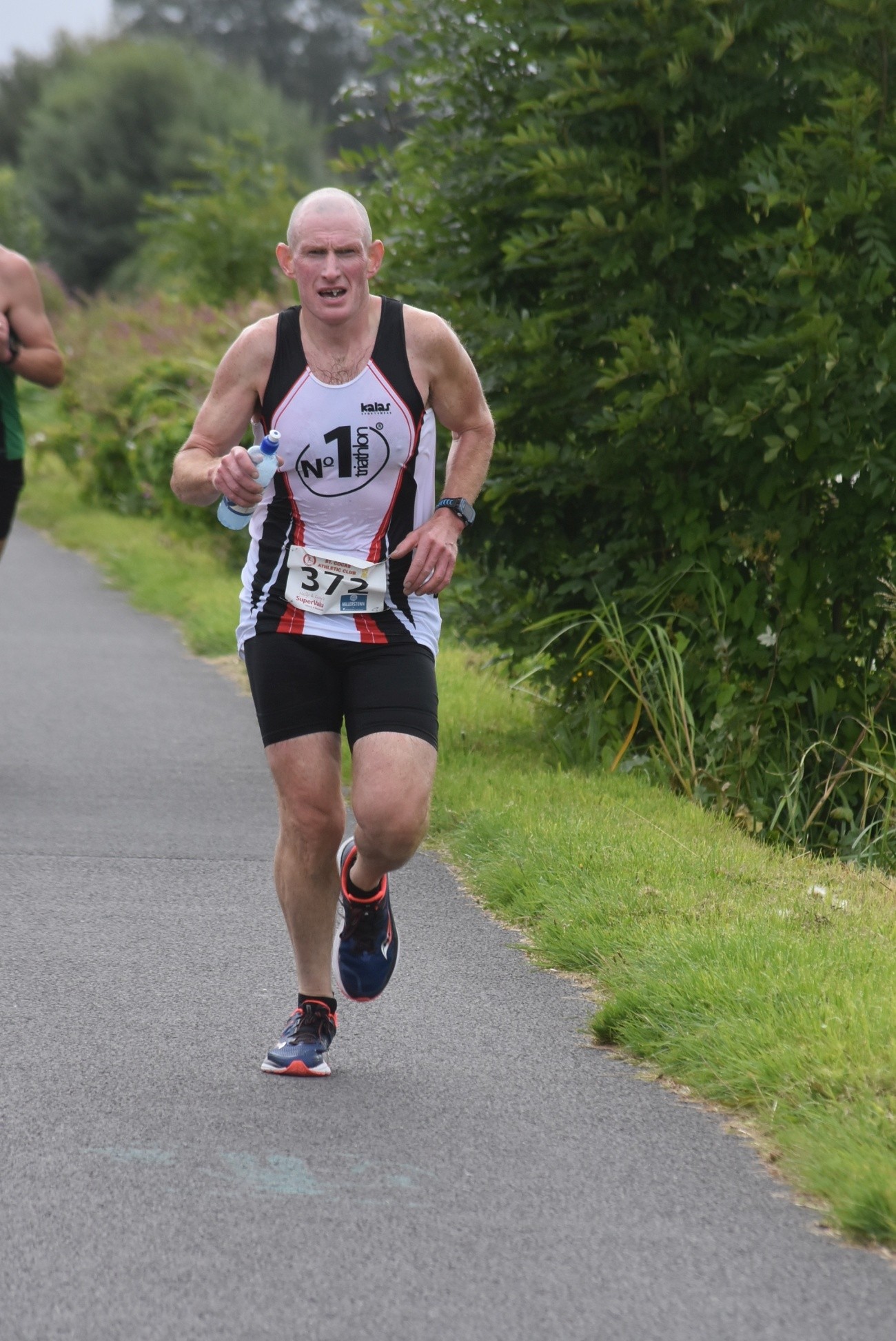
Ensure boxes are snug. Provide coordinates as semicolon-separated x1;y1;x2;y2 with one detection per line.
0;244;64;556
170;187;496;1077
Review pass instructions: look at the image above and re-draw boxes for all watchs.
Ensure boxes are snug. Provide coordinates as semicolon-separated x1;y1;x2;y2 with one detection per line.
434;497;476;527
1;338;19;365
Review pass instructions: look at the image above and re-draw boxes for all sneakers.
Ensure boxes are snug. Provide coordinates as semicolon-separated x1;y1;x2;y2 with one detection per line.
261;1001;337;1076
337;836;399;1003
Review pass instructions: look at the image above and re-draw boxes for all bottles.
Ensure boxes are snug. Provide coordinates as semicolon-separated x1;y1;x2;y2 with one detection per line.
216;430;281;531
